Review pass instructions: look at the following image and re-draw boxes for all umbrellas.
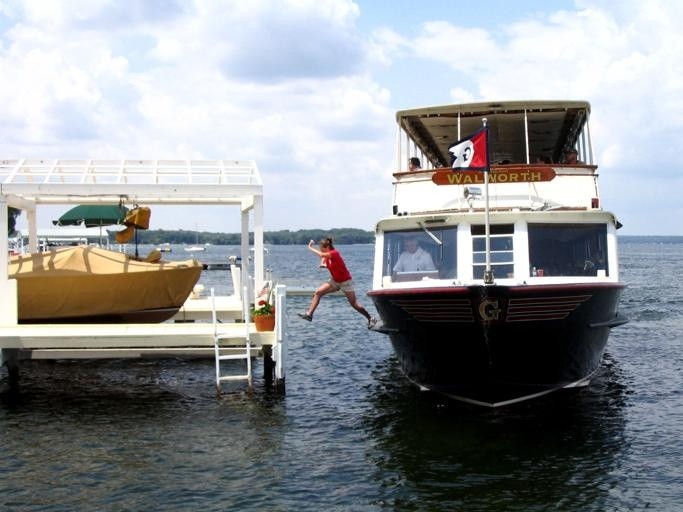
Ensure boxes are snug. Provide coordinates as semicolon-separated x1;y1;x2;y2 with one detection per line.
52;205;129;248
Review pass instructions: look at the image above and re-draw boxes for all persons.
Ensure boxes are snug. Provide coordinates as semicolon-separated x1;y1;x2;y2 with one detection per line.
393;237;436;272
409;158;423;171
298;238;377;329
566;149;585;164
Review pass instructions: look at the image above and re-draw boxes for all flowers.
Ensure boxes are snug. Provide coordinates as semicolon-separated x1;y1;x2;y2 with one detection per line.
250;286;276;317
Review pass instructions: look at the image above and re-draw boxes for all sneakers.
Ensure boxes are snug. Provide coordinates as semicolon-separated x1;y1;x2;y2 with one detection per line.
367;316;377;330
297;312;313;322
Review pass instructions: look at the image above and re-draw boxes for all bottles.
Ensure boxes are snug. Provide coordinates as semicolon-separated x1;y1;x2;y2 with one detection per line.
532;266;538;276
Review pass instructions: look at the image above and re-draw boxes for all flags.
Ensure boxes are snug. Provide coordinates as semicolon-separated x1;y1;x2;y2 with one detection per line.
448;127;489;172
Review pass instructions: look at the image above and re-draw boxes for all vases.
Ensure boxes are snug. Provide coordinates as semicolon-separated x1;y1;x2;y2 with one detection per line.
252;314;275;331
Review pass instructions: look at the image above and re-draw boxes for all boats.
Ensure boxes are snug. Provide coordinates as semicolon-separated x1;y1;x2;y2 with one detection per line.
367;100;629;418
